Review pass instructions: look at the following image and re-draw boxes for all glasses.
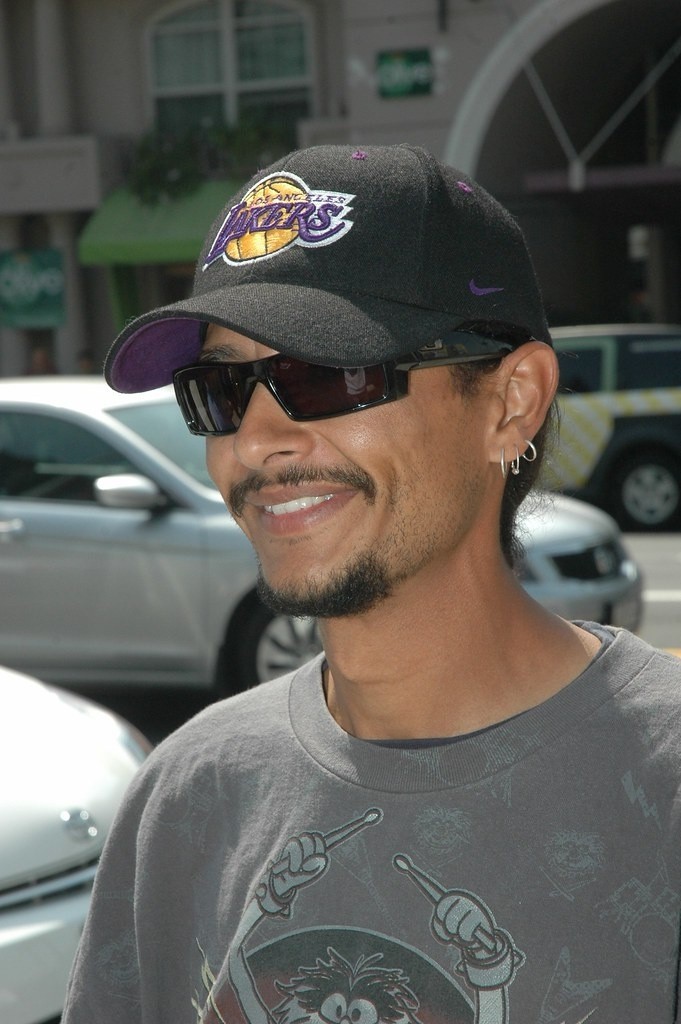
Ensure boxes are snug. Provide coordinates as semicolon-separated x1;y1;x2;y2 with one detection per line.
174;353;506;440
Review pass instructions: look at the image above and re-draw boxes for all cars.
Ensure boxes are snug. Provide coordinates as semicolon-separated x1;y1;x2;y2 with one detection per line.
529;320;681;540
1;368;648;719
0;656;171;1024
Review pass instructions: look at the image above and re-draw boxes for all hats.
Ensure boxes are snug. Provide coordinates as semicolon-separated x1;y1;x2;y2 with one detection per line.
103;144;553;394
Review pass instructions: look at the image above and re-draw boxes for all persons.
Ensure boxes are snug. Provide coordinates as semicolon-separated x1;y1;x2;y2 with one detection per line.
63;144;681;1024
